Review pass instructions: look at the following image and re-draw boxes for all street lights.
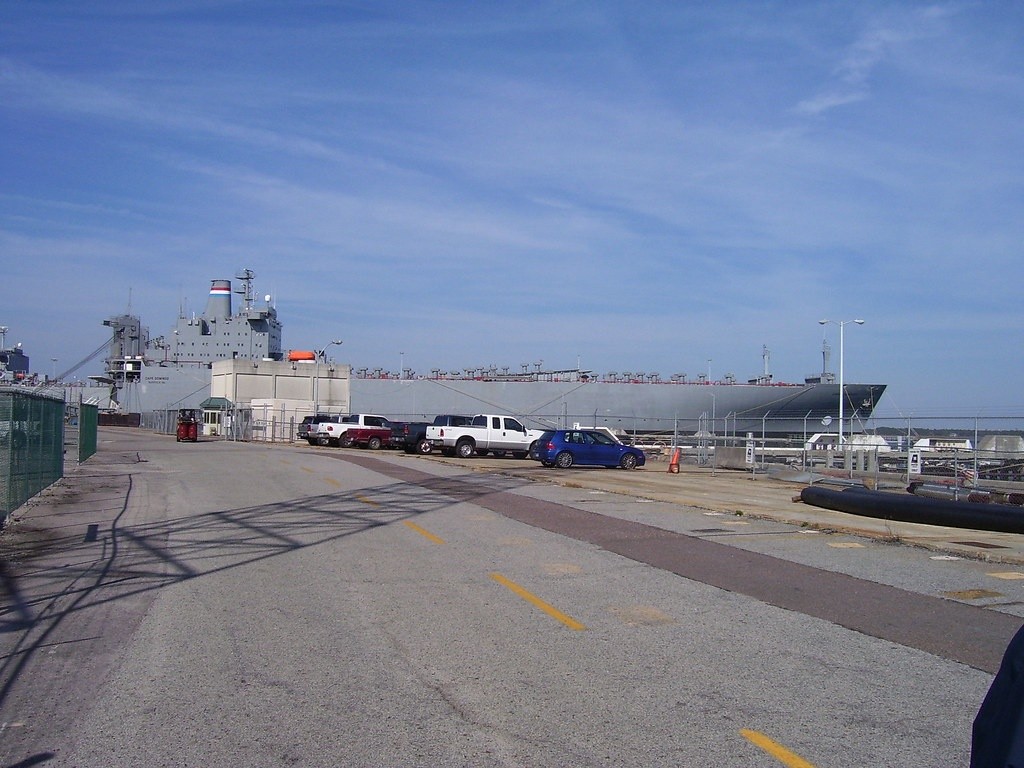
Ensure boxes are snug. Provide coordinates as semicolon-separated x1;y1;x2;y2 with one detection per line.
51;358;59;378
707;357;712;381
399;351;405;378
314;339;343;416
577;354;580;368
817;318;867;452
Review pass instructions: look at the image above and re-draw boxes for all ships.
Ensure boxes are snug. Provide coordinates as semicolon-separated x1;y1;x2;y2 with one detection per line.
0;265;888;446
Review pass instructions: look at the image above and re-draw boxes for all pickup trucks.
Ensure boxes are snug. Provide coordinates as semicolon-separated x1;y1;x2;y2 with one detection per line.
344;420;405;451
425;413;545;462
388;414;490;457
315;414;391;448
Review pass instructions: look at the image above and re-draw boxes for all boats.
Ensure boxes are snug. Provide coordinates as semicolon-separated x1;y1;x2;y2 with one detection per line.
288;350;315;361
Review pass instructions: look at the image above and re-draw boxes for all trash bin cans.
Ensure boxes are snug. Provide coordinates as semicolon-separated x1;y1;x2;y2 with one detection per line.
228;408;254;442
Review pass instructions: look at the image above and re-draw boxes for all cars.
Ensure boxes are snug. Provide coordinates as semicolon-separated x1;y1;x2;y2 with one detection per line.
536;428;646;471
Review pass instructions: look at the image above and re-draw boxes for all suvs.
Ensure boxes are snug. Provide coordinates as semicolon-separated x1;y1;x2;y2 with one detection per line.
300;414;352;447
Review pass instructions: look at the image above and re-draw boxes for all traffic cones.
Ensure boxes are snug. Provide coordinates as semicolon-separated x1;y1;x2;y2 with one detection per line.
670;449;681;474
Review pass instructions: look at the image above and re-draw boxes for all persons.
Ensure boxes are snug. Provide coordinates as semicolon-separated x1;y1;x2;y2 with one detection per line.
180;410;195;420
